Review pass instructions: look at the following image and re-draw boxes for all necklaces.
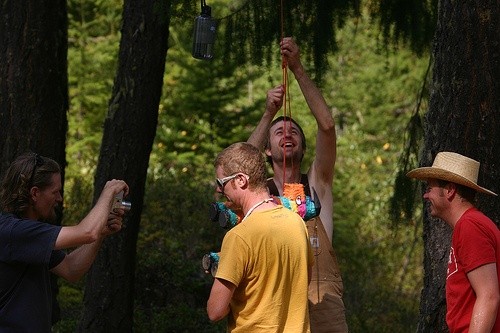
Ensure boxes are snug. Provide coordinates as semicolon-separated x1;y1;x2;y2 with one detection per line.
241;198;273;222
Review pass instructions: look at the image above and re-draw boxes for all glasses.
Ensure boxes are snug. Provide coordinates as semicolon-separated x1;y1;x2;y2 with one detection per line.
216;172;250;189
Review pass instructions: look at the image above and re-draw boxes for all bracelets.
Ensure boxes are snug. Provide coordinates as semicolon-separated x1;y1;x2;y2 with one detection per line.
296;66;304;80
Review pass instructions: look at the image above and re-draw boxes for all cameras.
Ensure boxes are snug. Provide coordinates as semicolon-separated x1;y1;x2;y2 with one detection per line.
109;190;132;214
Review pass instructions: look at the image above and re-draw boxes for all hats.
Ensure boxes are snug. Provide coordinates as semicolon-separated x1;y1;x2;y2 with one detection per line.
405;151;498;196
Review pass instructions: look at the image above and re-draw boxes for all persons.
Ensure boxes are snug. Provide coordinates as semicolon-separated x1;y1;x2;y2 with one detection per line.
406;151;500;333
246;37;348;333
0;154;129;333
207;143;311;333
